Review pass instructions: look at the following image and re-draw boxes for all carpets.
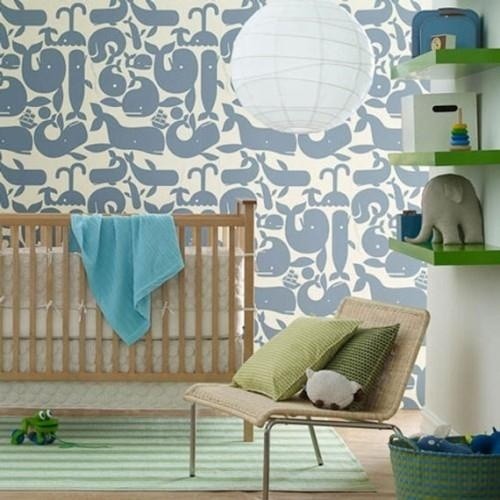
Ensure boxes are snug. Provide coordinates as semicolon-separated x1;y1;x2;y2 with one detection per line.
1;414;379;500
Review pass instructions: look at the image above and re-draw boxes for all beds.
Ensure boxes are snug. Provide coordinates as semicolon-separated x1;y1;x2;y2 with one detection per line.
0;200;253;441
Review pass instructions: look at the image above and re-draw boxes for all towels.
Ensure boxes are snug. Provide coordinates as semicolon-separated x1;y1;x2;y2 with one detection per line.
67;213;184;346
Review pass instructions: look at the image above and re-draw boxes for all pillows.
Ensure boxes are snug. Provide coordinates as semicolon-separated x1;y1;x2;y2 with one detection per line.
230;318;400;410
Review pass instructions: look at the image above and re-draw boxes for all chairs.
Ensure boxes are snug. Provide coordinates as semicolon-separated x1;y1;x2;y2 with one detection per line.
182;298;430;498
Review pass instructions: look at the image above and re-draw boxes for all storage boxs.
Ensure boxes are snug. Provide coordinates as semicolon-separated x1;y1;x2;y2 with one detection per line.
400;91;479;154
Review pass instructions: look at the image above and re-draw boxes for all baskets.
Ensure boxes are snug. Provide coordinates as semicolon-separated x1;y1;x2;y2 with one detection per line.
387;432;499;499
